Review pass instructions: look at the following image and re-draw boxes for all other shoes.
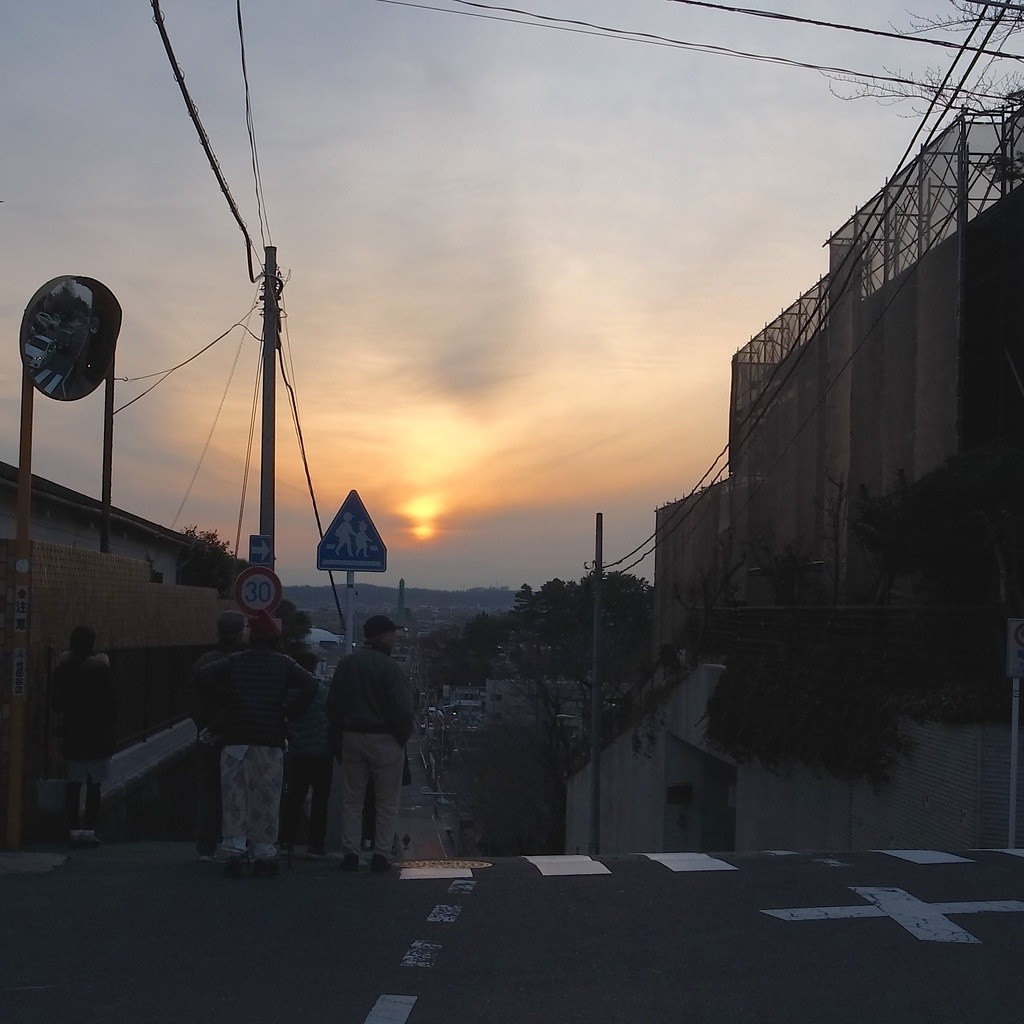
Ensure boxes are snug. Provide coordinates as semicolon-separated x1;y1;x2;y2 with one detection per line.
81;835;100;848
344;854;359;866
200;852;216;863
305;846;332;860
253;859;279;875
372;855;393;869
226;864;243;877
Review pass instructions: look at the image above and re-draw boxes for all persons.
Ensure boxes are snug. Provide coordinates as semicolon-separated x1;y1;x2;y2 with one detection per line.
51;624;118;849
182;598;414;877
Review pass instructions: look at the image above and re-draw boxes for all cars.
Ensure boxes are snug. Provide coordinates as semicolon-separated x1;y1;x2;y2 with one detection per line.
24;334;57;369
34;311;62;327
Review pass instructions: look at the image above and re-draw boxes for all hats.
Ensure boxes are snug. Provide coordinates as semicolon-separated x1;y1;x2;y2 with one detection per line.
364;615;404;636
218;610;244;632
249;609;281;642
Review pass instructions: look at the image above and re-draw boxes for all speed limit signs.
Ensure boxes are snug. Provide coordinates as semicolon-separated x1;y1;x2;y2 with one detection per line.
234;566;283;619
